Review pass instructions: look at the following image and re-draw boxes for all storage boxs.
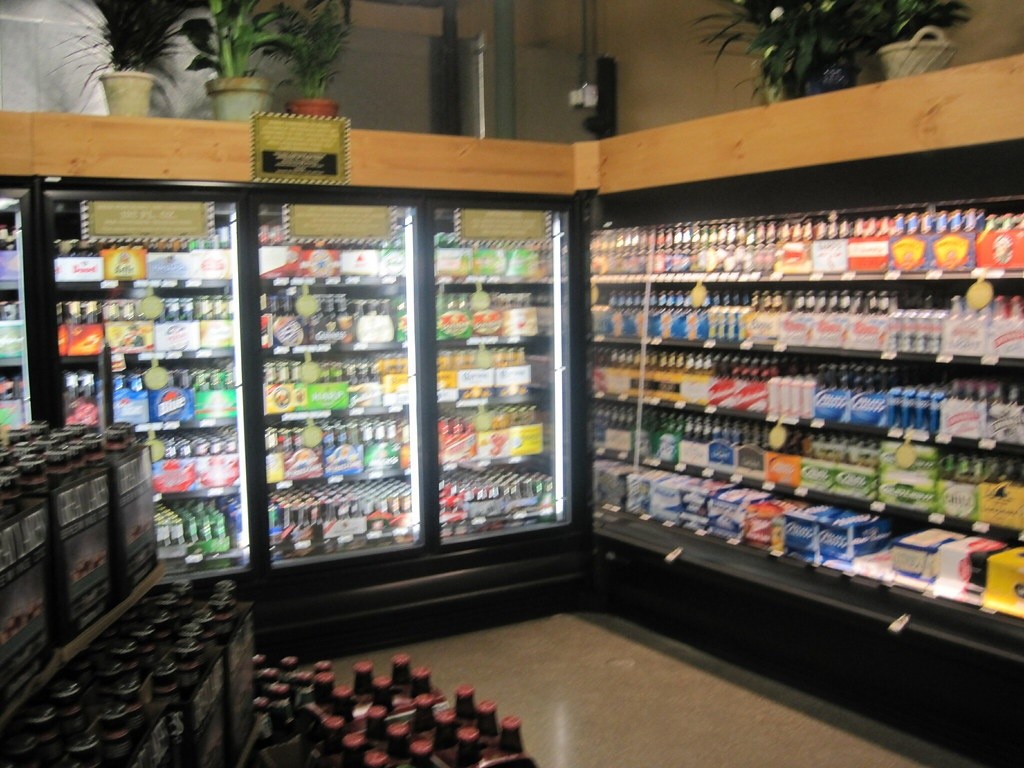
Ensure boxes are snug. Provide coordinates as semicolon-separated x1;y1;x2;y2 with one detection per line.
776;229;1024;275
0;245;545;768
593;441;1024;618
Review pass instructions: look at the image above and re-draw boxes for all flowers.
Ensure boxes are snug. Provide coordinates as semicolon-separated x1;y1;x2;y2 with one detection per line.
684;0;893;103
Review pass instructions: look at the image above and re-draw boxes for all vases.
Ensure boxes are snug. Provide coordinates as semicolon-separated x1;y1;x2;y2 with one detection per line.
778;64;861;97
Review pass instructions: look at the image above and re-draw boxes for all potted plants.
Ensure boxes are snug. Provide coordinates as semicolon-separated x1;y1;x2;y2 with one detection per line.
877;1;975;82
173;0;290;121
46;1;211;117
261;0;351;116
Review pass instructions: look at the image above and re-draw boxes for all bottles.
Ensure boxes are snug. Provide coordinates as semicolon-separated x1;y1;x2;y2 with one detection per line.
3;208;1023;767
